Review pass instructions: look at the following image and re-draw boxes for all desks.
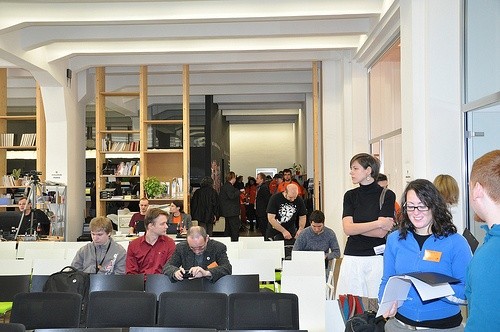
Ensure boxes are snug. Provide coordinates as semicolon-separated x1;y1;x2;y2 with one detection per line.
0;241;284;268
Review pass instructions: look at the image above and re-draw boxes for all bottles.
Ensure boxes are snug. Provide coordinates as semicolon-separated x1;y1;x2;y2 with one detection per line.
37;223;42;240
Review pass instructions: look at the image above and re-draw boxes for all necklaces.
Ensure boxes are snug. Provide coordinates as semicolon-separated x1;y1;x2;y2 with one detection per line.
95;240;112;273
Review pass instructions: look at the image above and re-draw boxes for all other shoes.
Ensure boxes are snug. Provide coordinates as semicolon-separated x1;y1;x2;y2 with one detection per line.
241;222;260;232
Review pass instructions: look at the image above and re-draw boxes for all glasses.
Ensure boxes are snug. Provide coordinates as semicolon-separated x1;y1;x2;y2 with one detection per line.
190;246;204;250
403;202;429;211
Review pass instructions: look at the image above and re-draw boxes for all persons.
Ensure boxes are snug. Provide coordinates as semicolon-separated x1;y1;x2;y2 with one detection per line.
290;210;340;279
191;176;221;237
1;174;36;186
377;174;401;239
128;197;151;235
377;174;472;332
166;200;192;233
89;177;140;231
162;225;232;285
432;175;462;236
101;139;140;151
69;216;127;274
17;197;50;236
0;133;36;147
463;150;500;332
342;153;404;315
266;184;306;242
126;208;176;284
219;169;305;242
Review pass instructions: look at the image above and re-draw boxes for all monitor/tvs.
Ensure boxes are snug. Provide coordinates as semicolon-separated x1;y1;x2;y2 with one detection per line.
0;211;25;234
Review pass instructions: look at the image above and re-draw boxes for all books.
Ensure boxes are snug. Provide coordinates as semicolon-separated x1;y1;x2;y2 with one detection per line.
112;159;140;175
375;271;462;318
161;178;183;198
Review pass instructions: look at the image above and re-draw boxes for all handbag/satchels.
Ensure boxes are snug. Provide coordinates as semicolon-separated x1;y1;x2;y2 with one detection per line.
344;310;386;332
379;189;400;239
43;266;90;328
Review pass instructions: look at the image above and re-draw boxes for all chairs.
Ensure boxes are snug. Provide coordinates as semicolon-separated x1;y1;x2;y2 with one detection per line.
2;237;328;332
212;216;226;233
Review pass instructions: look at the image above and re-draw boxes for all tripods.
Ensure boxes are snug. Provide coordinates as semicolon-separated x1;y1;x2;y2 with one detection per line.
13;180;59;241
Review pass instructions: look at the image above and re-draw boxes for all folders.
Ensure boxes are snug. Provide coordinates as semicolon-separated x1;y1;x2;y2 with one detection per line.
376;272;461;318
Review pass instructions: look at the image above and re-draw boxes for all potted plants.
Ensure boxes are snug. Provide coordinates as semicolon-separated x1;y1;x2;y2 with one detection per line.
289;162;303;175
143;177;162;199
10;169;24;187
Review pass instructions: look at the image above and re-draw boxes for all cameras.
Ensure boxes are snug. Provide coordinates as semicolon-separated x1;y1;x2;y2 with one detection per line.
181;270;193;277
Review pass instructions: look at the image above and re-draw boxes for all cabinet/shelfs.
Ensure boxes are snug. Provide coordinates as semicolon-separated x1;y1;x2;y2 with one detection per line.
31;184;68;241
0;69;230;212
